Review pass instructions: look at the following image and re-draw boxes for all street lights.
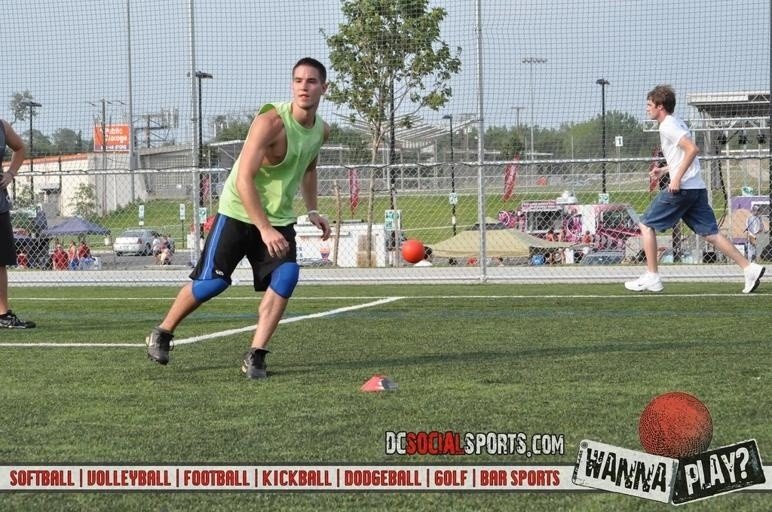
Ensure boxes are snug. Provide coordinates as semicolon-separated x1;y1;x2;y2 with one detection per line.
521;57;547;183
442;112;458;266
20;101;43;206
595;78;612;194
186;69;215;253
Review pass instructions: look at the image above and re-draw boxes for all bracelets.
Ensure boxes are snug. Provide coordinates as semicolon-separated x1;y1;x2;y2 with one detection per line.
7;169;16;179
307;208;321;215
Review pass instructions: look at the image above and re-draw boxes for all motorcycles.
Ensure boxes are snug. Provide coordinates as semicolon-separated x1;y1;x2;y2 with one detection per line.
386;235;408;250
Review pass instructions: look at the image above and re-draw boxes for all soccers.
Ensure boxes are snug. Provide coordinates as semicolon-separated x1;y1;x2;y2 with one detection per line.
639;393;712;458
402;240;425;264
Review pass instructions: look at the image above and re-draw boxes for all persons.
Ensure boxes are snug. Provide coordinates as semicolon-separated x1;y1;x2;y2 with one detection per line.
403;239;504;267
33;205;48;231
312;245;332;266
143;55;331;377
0;118;38;330
152;231;177;266
15;252;30;269
68;240;80;270
745;205;765;262
52;243;70;270
547;225;717;265
78;241;92;259
624;83;765;293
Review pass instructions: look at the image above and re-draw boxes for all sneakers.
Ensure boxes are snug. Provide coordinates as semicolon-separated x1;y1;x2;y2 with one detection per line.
742;262;765;294
0;309;36;330
241;346;269;380
625;273;664;293
145;327;175;365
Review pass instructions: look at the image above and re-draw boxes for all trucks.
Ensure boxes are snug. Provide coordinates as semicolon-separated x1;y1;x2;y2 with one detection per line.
497;190;642;264
701;195;769;263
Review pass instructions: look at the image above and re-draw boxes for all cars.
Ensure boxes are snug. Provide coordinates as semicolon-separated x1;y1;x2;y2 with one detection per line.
112;229;160;257
578;251;625;263
